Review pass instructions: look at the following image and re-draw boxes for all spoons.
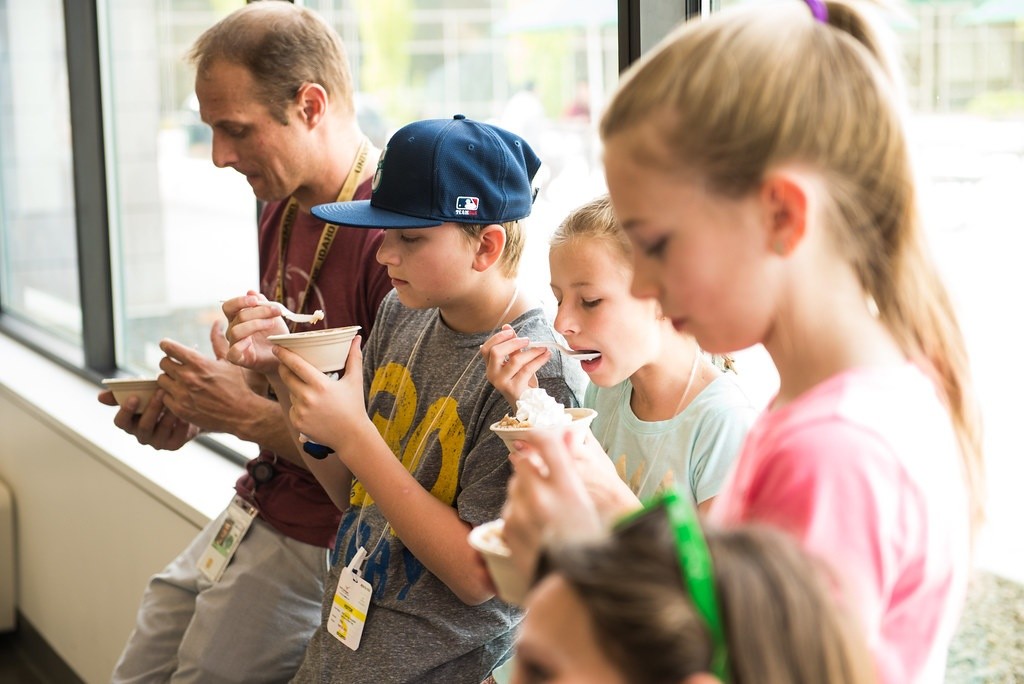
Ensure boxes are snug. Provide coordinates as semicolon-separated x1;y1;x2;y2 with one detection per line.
480;341;601;361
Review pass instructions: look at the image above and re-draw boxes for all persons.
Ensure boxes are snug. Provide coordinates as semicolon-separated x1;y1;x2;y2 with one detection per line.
98;0;394;684
509;493;872;684
480;192;755;684
222;114;592;684
605;0;984;684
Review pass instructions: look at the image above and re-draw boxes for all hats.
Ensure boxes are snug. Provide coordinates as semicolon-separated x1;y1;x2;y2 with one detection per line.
311;112;543;229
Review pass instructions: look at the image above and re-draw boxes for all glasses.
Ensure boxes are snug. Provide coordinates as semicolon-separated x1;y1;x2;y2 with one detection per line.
611;486;733;684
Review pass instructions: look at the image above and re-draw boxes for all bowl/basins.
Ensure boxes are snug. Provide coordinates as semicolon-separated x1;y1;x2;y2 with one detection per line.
101;377;160;415
266;325;362;372
489;407;597;453
467;518;522;603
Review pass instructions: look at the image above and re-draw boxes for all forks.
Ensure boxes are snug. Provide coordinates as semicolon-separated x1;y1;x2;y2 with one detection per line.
251;300;314;323
